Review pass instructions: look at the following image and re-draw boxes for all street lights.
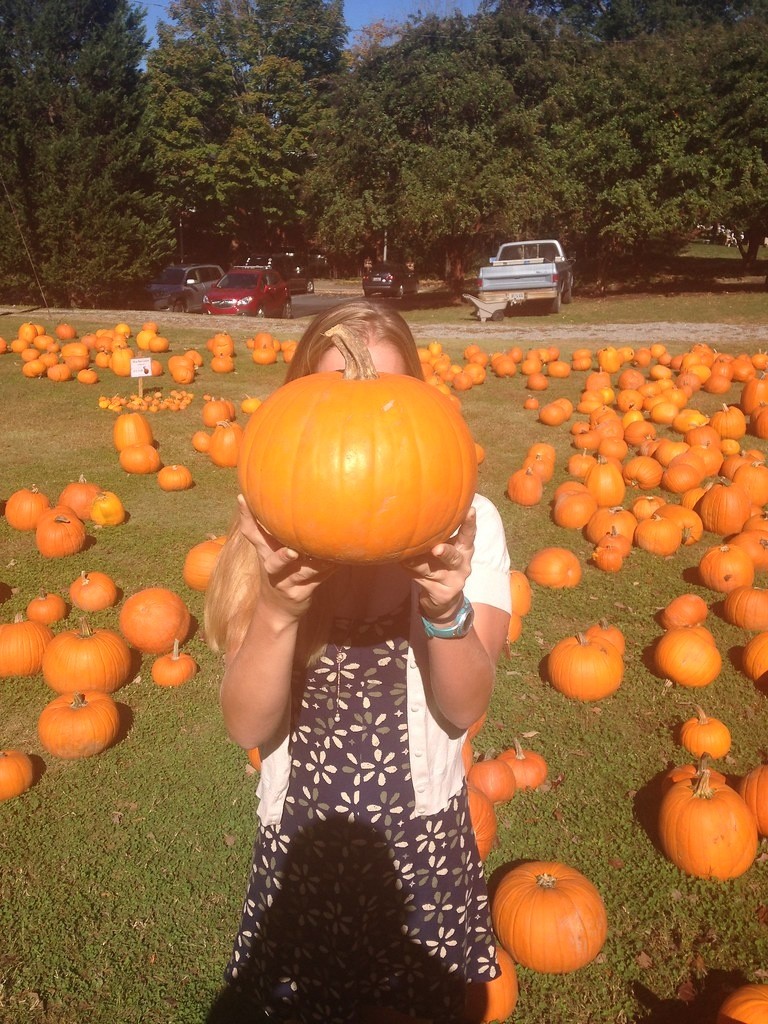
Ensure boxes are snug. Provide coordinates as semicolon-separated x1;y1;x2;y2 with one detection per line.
179;206;197;264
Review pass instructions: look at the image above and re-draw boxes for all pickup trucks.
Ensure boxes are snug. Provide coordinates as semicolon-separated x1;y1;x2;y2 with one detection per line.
477;238;577;313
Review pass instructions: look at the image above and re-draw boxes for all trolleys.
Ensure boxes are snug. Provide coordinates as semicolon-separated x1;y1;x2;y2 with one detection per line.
461;293;519;324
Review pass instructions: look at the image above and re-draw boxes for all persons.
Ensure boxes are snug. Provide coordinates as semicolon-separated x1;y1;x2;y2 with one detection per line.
205;298;511;1023
406;256;413;270
364;255;372;271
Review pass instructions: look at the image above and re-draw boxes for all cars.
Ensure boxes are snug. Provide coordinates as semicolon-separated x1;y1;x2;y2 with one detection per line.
362;260;420;299
201;264;292;319
128;263;228;314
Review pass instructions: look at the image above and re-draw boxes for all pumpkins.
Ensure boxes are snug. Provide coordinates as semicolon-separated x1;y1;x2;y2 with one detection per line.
236;325;476;568
417;338;768;1024
0;319;301;804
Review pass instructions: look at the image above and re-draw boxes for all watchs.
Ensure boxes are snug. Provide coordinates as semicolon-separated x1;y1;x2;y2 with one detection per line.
421;598;475;640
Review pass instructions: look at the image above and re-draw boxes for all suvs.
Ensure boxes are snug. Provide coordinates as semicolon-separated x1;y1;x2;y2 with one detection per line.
245;253;315;293
